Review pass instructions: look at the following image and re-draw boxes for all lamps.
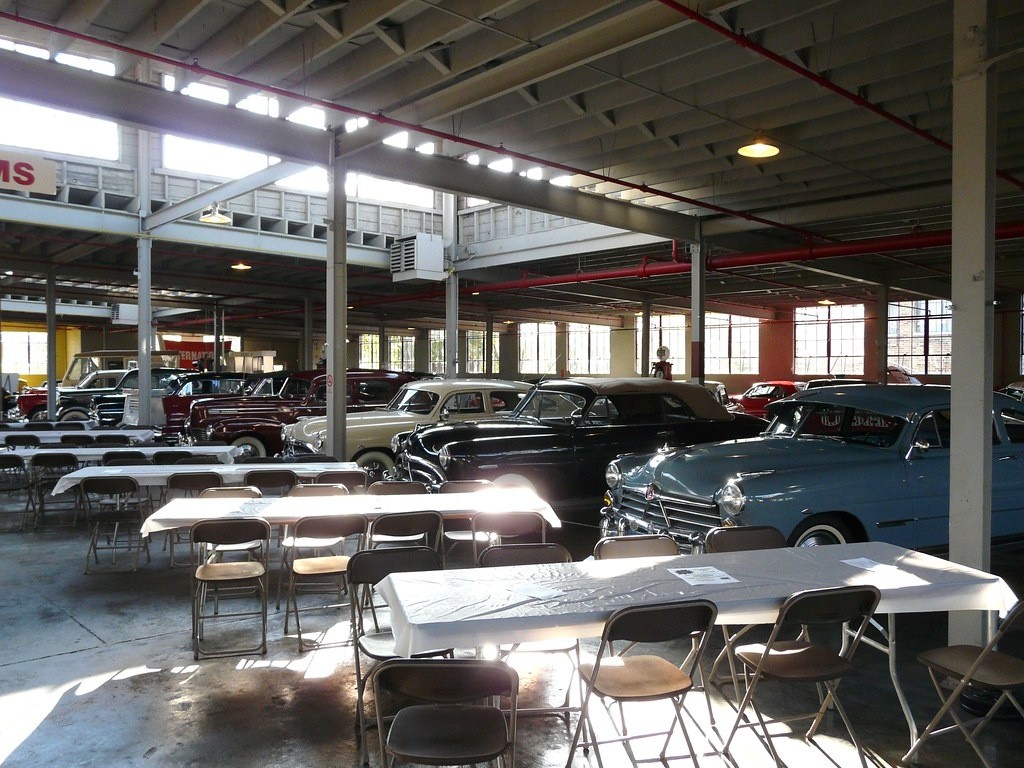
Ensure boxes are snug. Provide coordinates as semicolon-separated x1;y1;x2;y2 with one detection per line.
737;128;781;158
198;202;231;223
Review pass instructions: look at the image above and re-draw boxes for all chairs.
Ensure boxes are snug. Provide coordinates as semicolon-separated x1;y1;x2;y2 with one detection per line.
0;418;1024;768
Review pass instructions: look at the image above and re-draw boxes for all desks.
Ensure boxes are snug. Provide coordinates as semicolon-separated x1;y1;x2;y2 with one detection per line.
372;539;1019;768
140;496;563;642
50;464;368;565
0;445;240;522
4;420;100;430
0;429;155;483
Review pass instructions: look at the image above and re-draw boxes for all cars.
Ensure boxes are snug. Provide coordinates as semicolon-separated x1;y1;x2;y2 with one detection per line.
12;350;866;457
385;372;772;531
601;382;1024;559
277;377;595;481
